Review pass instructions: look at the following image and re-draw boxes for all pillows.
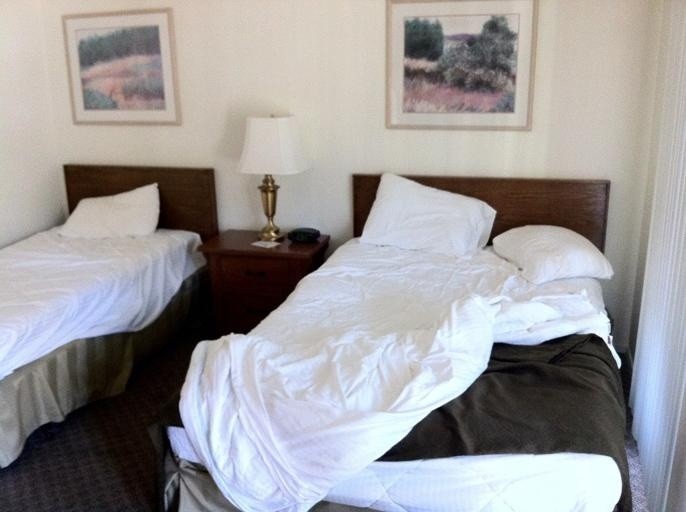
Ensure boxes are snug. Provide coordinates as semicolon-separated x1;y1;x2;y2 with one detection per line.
58;182;160;239
493;224;614;284
359;174;497;257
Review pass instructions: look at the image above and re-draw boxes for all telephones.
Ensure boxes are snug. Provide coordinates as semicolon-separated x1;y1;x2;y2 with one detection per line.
287;227;321;241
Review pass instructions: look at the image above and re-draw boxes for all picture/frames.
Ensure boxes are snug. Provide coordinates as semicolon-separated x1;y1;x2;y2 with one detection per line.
60;8;182;127
385;1;541;132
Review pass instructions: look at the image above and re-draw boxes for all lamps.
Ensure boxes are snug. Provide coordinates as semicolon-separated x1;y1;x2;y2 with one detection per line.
237;112;315;243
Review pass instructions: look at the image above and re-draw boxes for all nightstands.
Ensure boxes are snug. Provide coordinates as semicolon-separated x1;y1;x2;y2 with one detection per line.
196;230;331;336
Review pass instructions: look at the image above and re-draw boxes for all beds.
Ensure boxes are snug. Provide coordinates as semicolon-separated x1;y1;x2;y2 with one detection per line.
0;164;220;468
166;173;634;512
